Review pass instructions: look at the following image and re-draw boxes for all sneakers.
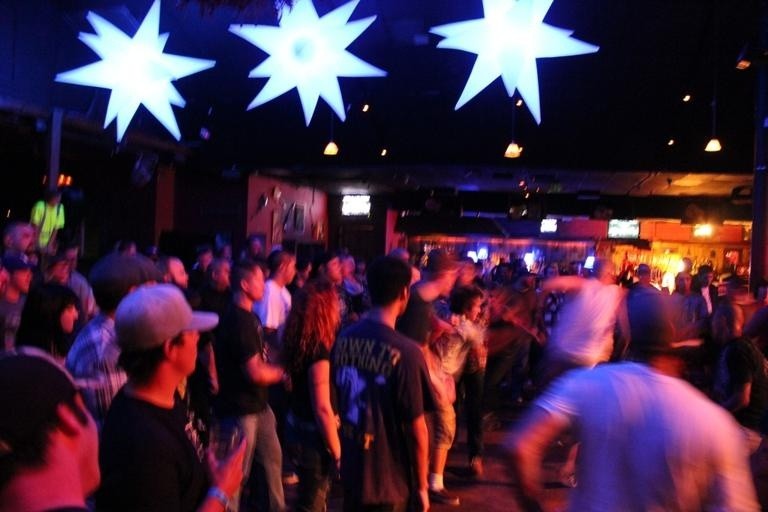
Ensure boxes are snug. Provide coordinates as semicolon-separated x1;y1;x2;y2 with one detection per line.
469;458;484;481
559;467;577;488
427;488;459;505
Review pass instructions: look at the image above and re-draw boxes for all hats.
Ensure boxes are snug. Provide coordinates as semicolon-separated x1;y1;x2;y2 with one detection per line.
113;284;220;353
88;252;156;284
421;249;461;276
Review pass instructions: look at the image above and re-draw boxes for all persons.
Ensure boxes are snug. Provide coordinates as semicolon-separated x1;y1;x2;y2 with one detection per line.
1;218;768;511
27;190;70;257
